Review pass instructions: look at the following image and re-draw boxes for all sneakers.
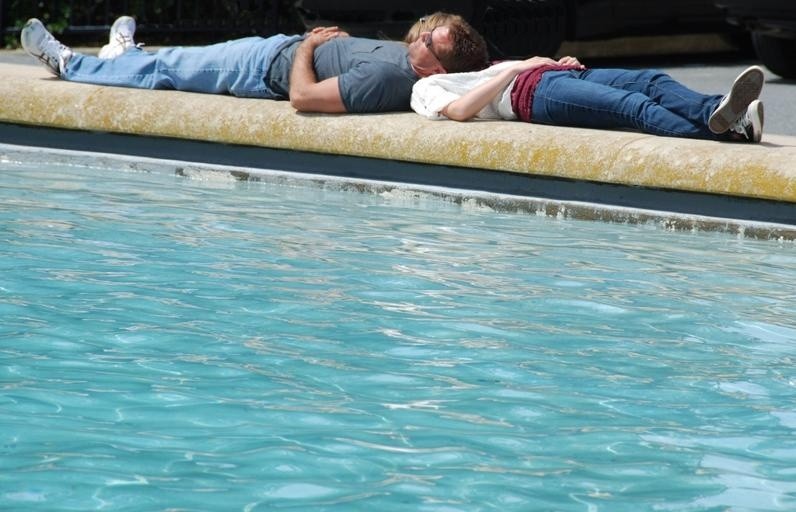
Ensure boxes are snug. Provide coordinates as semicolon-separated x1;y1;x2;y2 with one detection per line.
98;15;137;60
20;17;74;77
707;64;765;134
730;99;764;143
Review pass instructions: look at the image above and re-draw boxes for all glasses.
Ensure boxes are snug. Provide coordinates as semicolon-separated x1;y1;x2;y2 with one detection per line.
424;32;440;62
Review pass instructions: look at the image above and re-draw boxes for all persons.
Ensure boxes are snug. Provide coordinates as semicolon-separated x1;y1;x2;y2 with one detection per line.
404;10;766;145
18;13;490;117
